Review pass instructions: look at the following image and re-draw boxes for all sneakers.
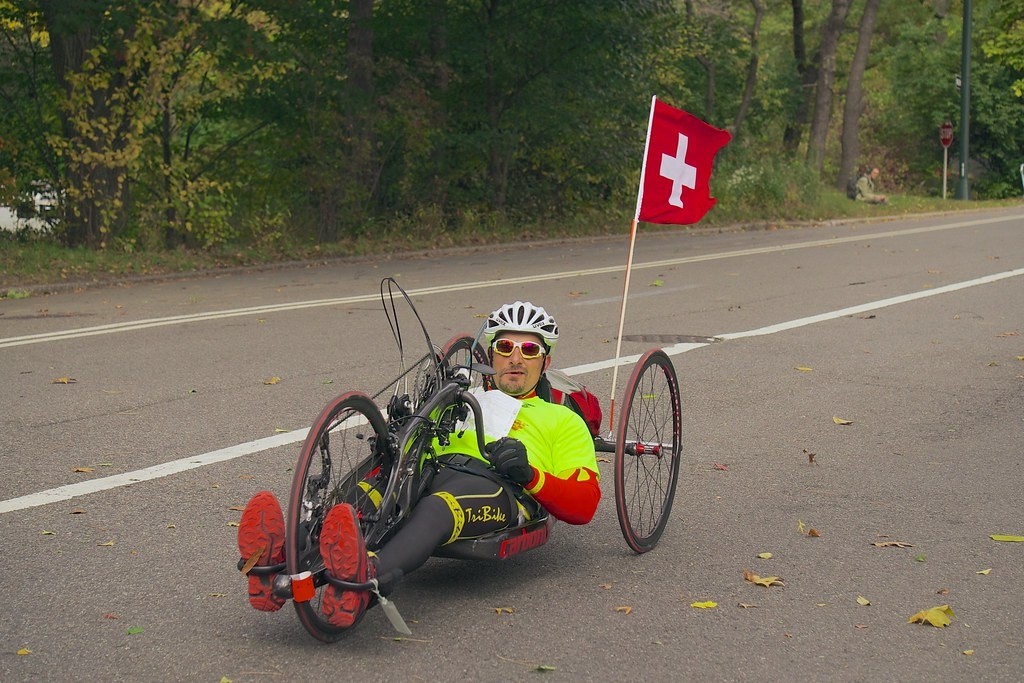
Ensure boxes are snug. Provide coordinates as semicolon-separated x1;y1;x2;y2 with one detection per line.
237;489;288;613
319;503;376;627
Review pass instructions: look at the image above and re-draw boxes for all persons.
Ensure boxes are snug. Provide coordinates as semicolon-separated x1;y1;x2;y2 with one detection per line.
237;299;602;629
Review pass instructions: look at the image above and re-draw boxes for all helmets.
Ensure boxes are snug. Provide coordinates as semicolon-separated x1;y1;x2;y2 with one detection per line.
484;301;559;356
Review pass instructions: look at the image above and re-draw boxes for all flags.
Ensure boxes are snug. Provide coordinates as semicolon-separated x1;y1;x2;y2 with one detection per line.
628;94;734;226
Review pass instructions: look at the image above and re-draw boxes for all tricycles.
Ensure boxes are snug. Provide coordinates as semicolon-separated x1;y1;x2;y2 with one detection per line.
236;277;683;644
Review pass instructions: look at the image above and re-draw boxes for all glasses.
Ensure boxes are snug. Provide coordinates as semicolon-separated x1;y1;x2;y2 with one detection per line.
492;339;546;359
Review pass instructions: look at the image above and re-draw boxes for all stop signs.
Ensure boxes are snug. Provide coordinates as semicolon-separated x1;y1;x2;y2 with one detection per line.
939;120;954;147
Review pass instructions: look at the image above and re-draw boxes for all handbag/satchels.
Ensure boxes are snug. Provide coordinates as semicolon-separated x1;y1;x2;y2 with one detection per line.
545;369;603;437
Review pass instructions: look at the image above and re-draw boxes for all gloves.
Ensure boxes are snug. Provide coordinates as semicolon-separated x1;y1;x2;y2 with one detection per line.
370;432;400;468
484;437;534;486
